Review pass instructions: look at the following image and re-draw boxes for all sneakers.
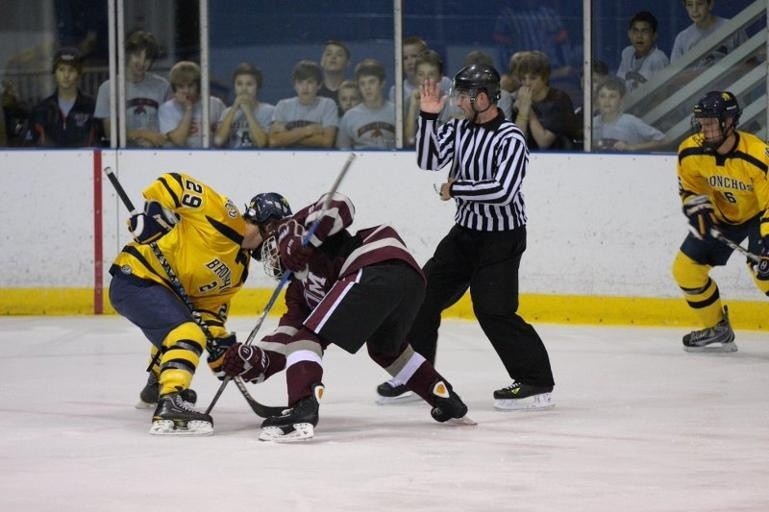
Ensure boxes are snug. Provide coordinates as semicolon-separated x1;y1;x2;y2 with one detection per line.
141;373;196;406
683;323;735;346
377;377;410;397
261;398;318;433
153;392;214;427
431;399;467;422
493;381;552;399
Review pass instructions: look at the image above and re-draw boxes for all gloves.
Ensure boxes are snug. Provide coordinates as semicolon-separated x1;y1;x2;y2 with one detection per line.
209;331;240;381
128;201;180;245
221;345;270;383
280;235;311;273
753;234;769;280
684;195;720;239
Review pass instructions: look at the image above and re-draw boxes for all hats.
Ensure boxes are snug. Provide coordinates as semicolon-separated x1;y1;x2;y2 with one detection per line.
52;48;82;74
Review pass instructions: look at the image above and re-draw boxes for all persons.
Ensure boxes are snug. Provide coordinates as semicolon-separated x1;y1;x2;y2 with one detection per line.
572;64;610;138
92;31;173;146
157;62;226;149
267;61;340;149
670;1;749;70
214;63;274;149
591;76;669;152
672;91;769;348
221;192;468;435
28;46;104;147
317;38;575;153
376;63;555;399
2;80;35;147
616;11;670;91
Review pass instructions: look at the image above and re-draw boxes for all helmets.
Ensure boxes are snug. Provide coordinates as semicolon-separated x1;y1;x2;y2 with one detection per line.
455;64;501;102
251;235;281;279
244;192;293;223
691;90;740;153
